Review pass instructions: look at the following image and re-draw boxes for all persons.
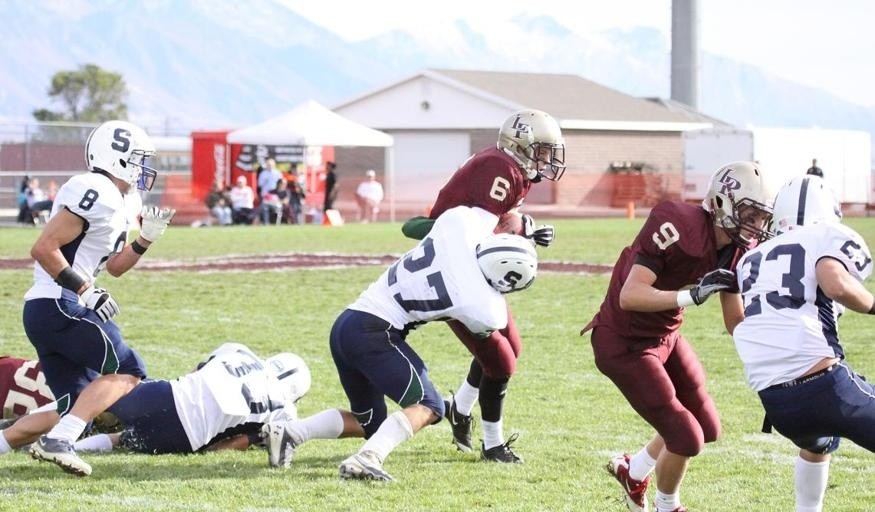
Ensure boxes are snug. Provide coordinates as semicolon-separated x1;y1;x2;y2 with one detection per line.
578;158;779;511
0;352;57;436
0;120;178;478
353;168;383;222
806;157;823;177
260;204;540;480
426;105;567;469
190;151;340;226
730;172;875;512
69;342;317;450
13;172;59;225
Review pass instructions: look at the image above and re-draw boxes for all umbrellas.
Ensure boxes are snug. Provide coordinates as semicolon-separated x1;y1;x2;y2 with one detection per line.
227;96;395;154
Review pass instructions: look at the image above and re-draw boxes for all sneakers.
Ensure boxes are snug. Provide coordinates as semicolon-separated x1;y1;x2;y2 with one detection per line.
444;398;519;462
262;420;298;468
29;434;92;475
607;455;649;512
339;453;391;480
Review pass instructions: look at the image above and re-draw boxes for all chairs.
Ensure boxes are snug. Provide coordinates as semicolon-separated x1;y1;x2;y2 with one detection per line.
13;182;50;225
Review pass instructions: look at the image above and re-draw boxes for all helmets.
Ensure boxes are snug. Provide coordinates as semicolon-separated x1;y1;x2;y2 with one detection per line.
85;120;156;190
497;110;566;181
702;162;842;250
268;352;311;402
477;232;538;292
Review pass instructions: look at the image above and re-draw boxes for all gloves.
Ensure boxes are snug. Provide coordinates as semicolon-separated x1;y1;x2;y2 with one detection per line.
138;206;176;242
80;285;119;323
690;269;739;305
533;225;554;246
